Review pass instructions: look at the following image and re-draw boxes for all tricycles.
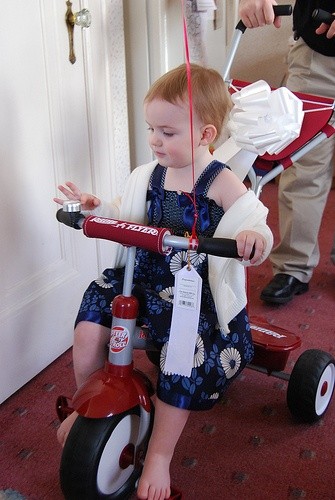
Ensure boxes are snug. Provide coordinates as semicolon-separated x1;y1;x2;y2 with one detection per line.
55;2;333;499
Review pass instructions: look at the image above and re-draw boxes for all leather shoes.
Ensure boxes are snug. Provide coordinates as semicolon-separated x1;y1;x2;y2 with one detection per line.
260;271;308;303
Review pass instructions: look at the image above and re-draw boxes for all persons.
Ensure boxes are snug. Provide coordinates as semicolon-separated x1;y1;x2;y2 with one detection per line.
236;0;335;308
46;60;276;500
